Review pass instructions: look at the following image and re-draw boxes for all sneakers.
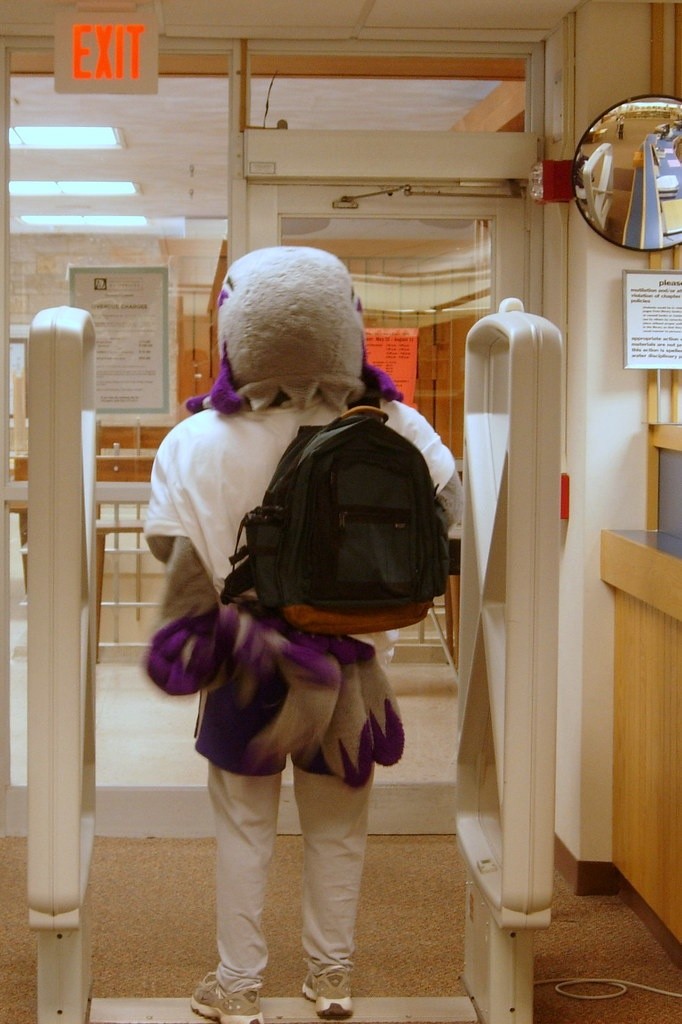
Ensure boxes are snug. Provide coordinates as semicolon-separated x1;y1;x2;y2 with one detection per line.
302;957;353;1017
191;971;264;1024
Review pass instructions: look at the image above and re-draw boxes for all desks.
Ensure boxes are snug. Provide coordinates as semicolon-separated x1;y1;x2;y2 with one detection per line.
5;427;173;662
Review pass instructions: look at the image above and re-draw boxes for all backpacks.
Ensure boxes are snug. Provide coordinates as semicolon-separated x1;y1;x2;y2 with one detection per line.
220;378;450;634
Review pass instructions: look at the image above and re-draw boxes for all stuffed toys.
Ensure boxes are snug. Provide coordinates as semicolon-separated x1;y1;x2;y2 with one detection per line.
142;248;464;1023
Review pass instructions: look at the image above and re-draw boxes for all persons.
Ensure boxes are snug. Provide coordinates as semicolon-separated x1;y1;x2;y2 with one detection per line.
618;115;624;139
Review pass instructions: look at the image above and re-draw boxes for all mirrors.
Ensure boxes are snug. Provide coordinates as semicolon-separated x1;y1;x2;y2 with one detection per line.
572;94;681;251
